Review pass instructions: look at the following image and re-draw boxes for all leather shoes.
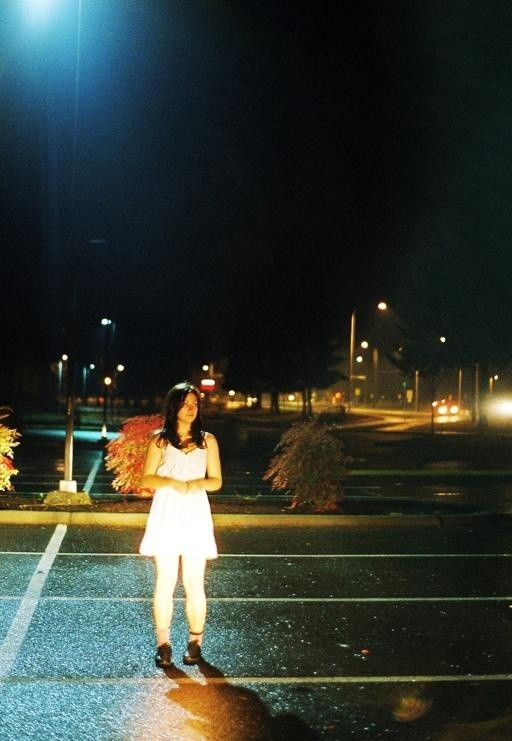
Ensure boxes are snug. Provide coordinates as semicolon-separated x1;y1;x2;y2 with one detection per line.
156;643;172;667
183;640;201;664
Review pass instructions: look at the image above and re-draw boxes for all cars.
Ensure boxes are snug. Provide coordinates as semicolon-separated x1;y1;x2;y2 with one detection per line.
431;399;459;416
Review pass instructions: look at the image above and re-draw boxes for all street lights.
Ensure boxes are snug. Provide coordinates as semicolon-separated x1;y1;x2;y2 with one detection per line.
430;336;448;434
489;371;499;398
370;298;389;408
54;313;127;493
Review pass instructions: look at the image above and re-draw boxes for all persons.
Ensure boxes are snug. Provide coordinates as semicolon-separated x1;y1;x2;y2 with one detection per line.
137;381;224;669
